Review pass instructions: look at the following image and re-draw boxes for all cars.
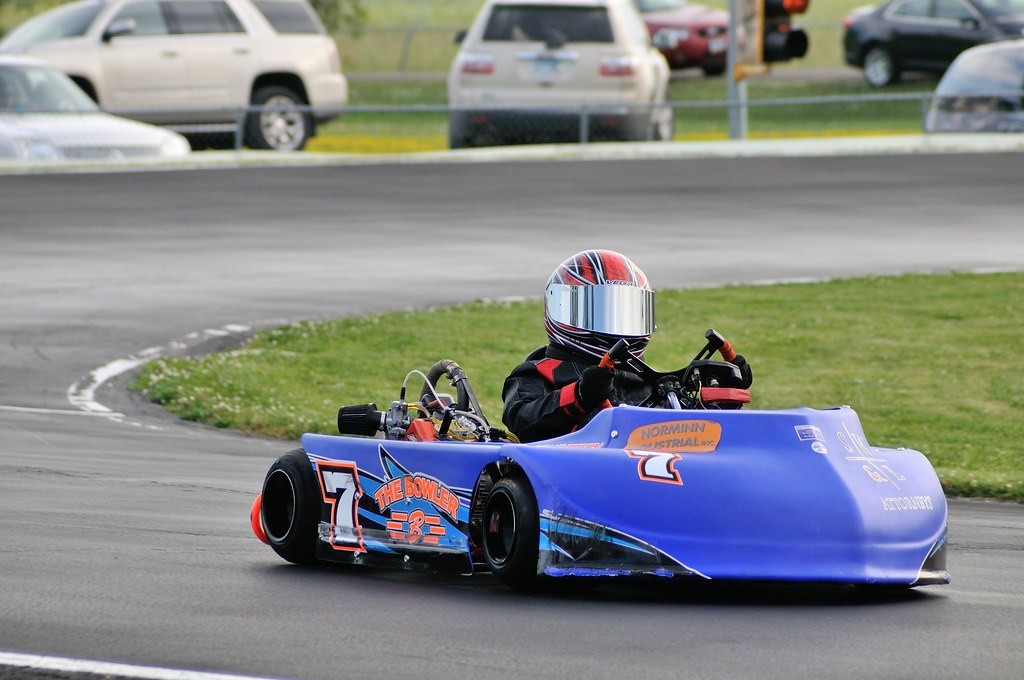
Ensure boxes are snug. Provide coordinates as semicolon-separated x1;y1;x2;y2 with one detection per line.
631;0;729;78
0;52;191;169
843;0;1024;90
924;39;1024;136
446;0;676;149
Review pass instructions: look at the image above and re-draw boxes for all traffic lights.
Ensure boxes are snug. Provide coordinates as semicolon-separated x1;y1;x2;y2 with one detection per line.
751;0;810;67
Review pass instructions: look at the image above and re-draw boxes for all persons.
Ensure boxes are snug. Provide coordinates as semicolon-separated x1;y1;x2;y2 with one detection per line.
502;249;753;443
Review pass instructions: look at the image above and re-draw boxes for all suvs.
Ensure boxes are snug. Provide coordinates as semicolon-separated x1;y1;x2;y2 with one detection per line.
0;0;349;152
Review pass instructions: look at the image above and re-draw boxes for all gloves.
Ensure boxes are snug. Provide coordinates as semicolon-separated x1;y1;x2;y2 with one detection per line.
576;366;618;412
730;353;752;390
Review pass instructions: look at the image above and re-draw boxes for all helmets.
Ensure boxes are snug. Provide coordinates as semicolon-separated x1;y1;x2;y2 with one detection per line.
544;250;655;363
